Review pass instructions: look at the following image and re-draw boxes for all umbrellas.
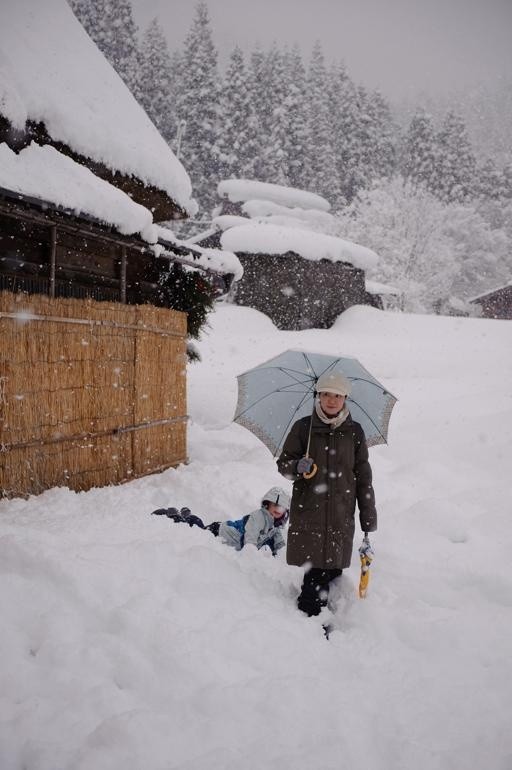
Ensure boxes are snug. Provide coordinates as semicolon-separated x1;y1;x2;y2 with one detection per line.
231;347;398;479
358;532;374;598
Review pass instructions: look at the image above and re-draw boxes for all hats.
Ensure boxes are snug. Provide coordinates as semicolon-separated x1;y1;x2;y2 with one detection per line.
315;371;352;396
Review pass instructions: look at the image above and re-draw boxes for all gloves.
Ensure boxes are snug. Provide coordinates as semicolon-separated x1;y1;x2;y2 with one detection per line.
297;456;314;475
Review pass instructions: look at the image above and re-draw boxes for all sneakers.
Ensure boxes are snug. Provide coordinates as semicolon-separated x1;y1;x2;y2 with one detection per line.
153;507;192;518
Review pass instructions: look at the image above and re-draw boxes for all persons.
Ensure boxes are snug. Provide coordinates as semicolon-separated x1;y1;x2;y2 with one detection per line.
275;374;377;619
151;482;291;556
